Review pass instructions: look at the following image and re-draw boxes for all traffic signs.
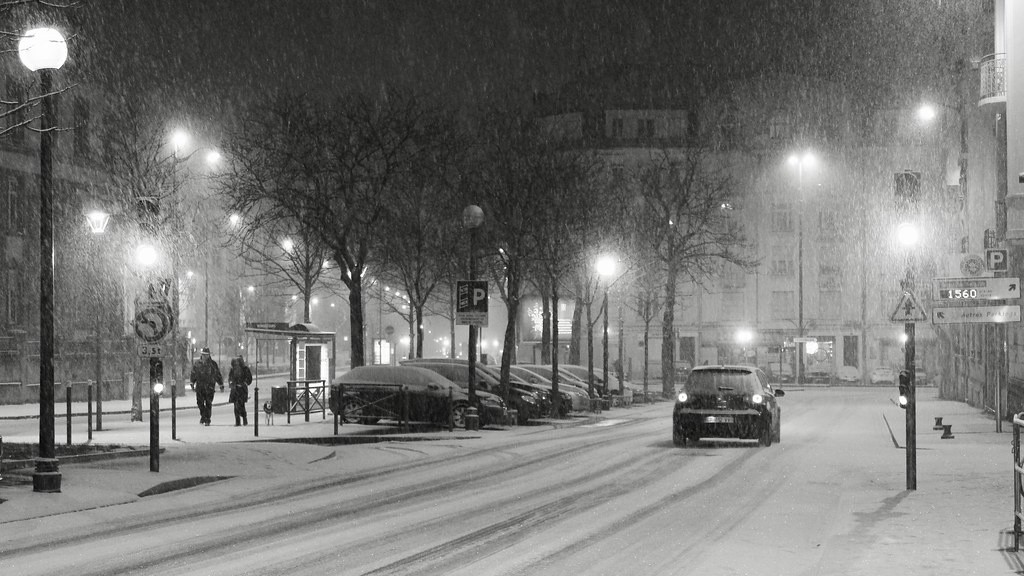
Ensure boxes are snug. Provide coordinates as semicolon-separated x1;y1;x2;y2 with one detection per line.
931;277;1020;325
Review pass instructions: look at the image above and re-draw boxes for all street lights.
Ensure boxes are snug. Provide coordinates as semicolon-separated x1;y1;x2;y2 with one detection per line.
462;206;485;432
919;100;968;250
172;133;254;440
897;221;922;490
85;209;109;432
19;26;67;492
599;253;617;394
789;151;816;384
136;244;161;473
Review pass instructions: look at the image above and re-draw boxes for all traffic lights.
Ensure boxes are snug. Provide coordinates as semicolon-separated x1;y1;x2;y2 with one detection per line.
899;368;911;408
152;358;165;396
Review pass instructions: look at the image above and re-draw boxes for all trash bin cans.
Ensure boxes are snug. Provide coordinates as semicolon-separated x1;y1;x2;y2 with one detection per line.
271;386;287;415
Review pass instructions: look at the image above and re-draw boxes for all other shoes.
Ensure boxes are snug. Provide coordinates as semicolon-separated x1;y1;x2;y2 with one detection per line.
243;417;247;425
199;416;205;424
236;417;240;425
205;418;210;426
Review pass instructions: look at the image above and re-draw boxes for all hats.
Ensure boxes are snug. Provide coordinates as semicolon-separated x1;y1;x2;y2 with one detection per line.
200;347;210;355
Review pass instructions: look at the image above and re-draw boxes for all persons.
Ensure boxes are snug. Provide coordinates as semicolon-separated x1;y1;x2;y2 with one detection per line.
228;355;252;426
190;347;224;426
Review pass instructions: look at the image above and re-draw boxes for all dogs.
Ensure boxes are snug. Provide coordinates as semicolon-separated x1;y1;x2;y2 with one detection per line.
263;400;273;425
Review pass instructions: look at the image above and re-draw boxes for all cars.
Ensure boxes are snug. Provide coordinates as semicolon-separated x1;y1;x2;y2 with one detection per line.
673;365;785;447
329;365;508;428
399;359;645;426
